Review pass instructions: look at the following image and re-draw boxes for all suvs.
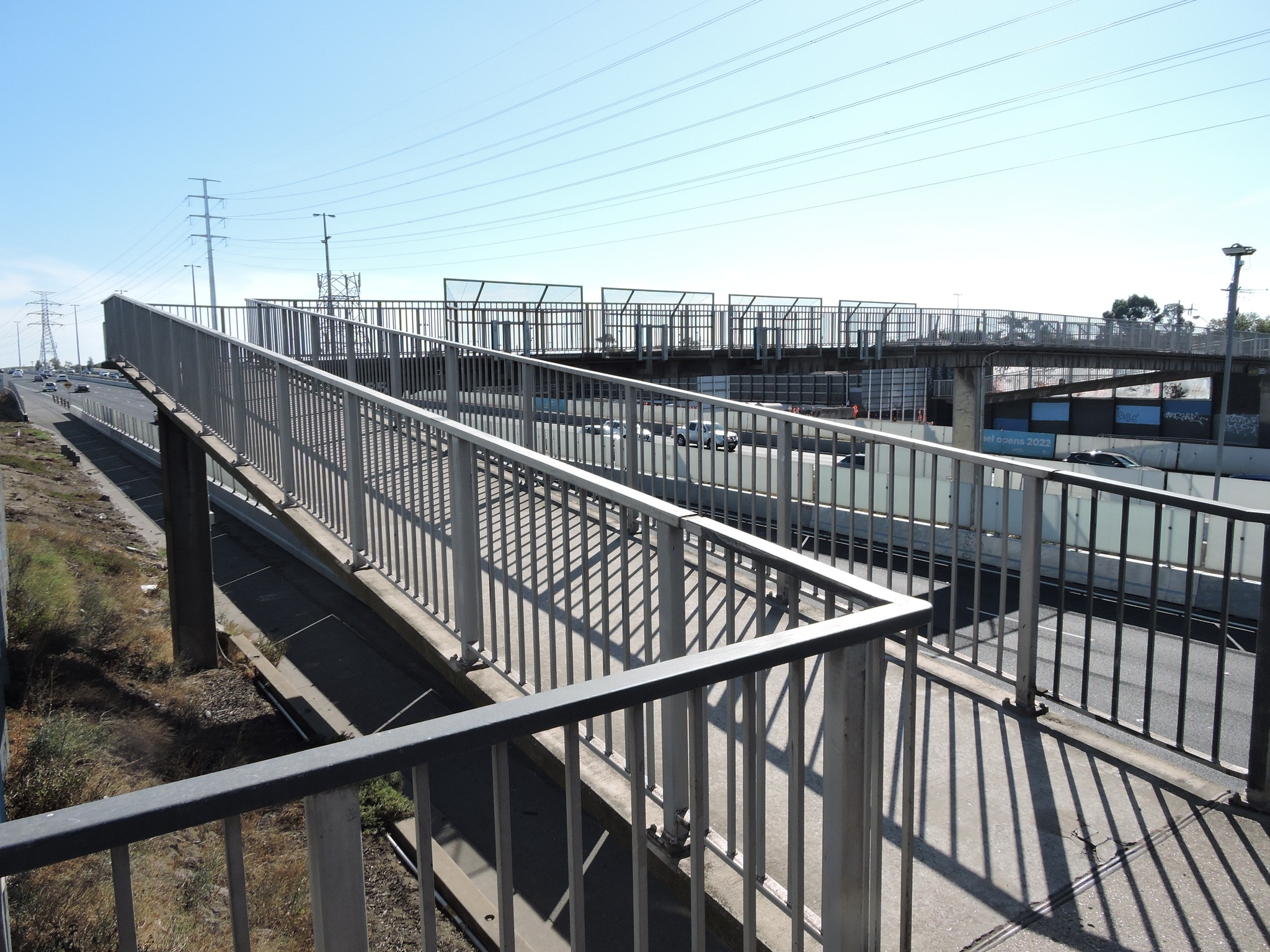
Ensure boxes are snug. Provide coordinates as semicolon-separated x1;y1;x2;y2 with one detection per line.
1061;449;1165;472
669;420;738;452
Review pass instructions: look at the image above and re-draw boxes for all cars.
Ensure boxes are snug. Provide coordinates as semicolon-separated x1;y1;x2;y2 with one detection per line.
154;410;159;425
31;375;44;382
603;419;651;442
8;368;16;375
38;367;57;380
0;369;4;373
118;372;125;379
74;383;90;393
82;369;91;375
57;368;66;373
56;374;69;383
41;382;57;392
66;368;75;373
99;371;109;377
15;369;23;375
577;424;624;440
12;371;22;378
109;372;119;379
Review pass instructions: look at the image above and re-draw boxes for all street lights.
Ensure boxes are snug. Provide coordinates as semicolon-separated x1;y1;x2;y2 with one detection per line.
70;304;82;376
313;213;336;316
14;321;22;369
114;290;128;295
184;264;202;323
